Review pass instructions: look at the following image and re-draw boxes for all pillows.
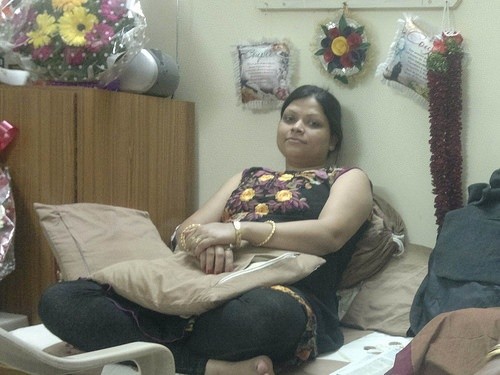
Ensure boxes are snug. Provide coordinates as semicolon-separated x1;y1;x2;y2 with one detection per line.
230;40;295;110
87;240;326;318
340;196;407;286
340;242;433;334
375;14;471;109
33;202;173;280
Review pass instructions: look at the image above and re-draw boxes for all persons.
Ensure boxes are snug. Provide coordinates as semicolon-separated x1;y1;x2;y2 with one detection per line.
37;85;374;375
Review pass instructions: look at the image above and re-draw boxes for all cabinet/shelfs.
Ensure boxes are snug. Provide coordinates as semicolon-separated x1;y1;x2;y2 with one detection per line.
0;85;196;325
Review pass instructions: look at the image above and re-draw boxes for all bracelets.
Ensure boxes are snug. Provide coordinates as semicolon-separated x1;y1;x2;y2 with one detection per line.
180;223;202;257
250;220;276;247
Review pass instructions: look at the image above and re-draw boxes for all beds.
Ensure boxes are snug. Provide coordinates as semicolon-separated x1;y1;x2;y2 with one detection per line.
10;233;434;375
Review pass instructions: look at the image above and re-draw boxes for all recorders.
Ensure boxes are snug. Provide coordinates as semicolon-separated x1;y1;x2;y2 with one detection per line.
105;0;181;97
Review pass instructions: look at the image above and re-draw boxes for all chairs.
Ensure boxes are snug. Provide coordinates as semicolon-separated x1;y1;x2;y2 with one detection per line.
0;329;175;374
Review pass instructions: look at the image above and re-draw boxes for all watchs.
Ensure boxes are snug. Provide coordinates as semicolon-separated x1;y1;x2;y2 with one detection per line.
226;219;241;249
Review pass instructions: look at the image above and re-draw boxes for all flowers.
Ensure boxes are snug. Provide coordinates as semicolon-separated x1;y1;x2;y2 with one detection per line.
314;15;371;85
0;0;149;86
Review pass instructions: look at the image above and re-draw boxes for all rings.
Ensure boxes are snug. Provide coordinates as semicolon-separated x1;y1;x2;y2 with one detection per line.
194;237;202;244
